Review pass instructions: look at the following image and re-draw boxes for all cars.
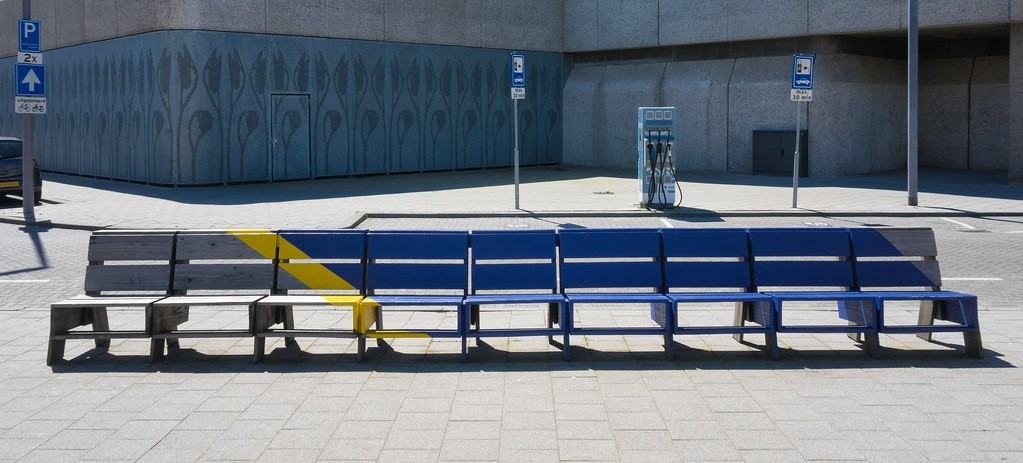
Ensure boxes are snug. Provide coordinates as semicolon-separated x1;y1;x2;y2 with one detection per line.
0;136;43;205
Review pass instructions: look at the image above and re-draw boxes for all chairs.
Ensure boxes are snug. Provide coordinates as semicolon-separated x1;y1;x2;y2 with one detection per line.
47;229;186;366
463;230;566;363
149;228;294;367
732;227;877;361
838;224;983;362
657;227;775;360
554;228;673;361
359;230;465;367
254;228;362;365
